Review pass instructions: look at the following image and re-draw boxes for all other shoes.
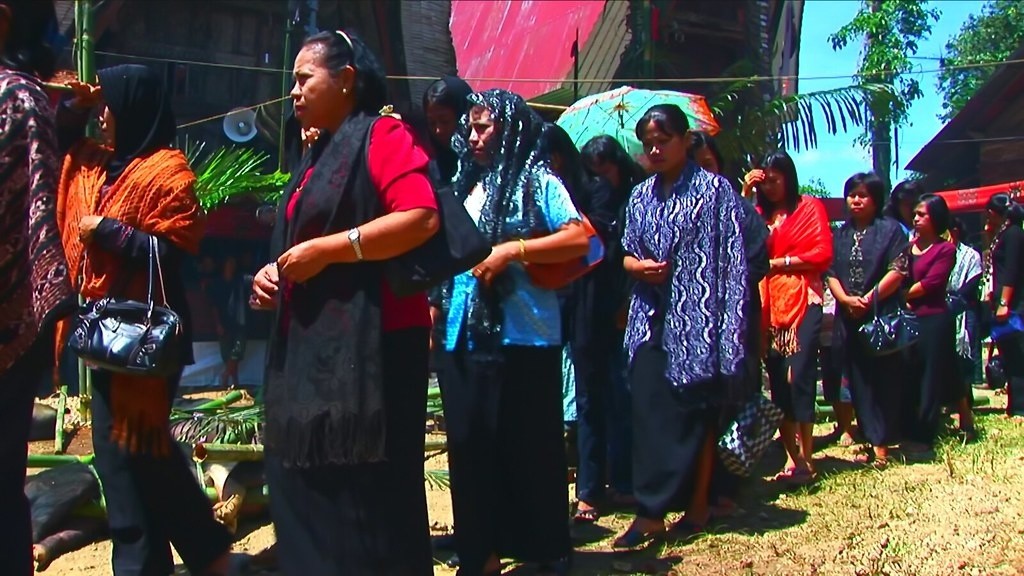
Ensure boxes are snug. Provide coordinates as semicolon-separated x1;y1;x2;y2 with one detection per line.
669;513;714;536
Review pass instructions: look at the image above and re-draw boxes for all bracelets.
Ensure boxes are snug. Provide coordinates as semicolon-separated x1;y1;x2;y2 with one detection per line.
519;239;530;262
785;256;791;272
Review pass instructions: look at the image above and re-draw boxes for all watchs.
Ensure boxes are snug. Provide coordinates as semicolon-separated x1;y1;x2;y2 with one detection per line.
348;228;365;260
1000;299;1008;306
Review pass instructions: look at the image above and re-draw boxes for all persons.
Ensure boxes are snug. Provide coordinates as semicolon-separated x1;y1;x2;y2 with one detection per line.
49;62;247;576
416;78;1024;576
0;1;74;575
248;30;434;575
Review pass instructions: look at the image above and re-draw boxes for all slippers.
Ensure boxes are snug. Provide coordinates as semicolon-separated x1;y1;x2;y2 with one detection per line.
575;503;599;523
795;466;820;484
614;527;666;547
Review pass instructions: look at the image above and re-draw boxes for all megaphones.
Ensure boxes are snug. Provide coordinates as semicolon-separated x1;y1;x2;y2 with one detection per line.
224;106;257;141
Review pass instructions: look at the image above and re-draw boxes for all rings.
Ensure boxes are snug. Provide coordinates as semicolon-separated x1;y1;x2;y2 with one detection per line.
657;270;664;275
477;269;483;274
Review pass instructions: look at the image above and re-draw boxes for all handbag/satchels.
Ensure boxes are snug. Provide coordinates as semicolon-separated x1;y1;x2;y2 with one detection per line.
523;209;604;289
364;114;493;296
986;339;1006;389
68;236;180;377
863;281;920;355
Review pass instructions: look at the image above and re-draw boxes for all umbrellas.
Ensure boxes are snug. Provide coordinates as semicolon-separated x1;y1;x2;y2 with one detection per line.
554;86;720;160
